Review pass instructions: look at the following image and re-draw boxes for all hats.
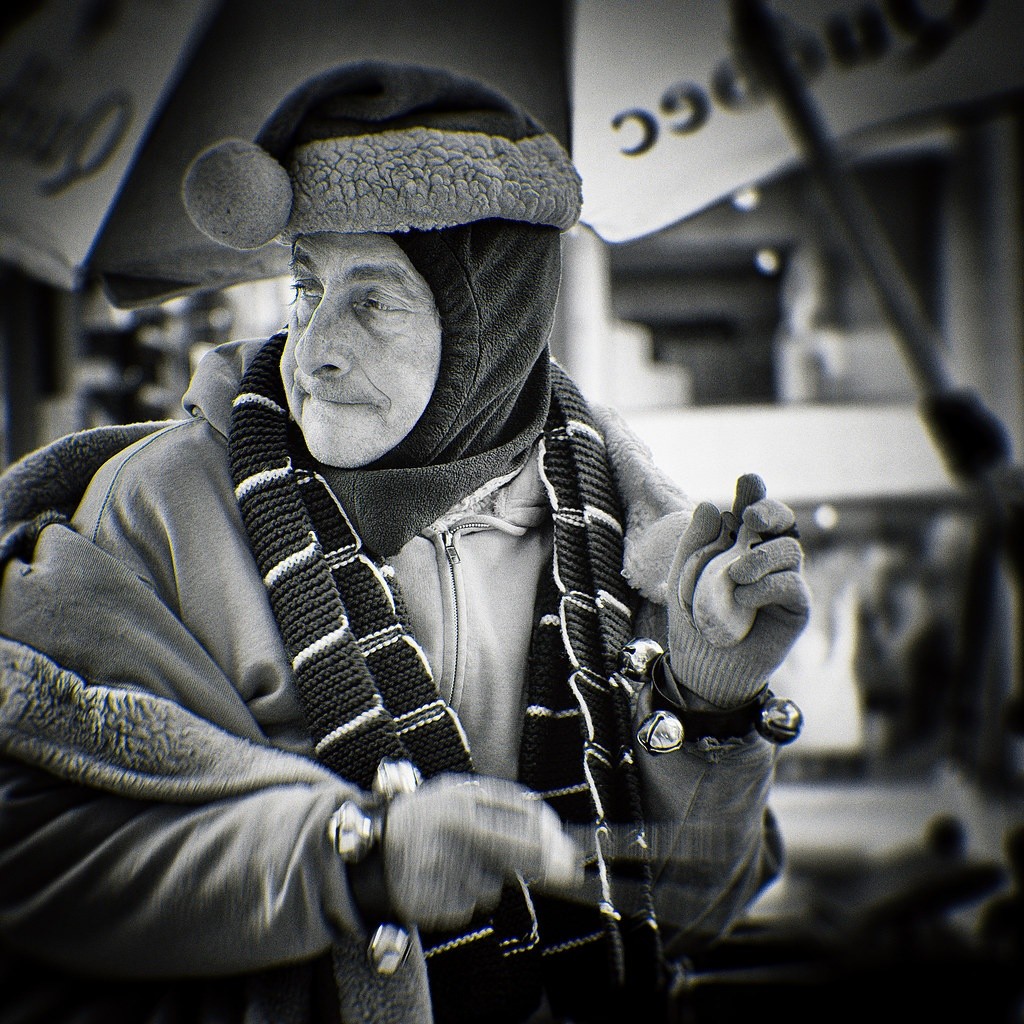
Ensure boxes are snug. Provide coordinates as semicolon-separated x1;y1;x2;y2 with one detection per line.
179;58;584;250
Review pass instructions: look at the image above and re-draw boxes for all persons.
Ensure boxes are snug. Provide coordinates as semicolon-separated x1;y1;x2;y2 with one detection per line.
0;59;811;1024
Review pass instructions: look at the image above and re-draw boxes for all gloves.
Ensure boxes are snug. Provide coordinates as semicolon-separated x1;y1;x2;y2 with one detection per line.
667;473;811;708
387;768;583;932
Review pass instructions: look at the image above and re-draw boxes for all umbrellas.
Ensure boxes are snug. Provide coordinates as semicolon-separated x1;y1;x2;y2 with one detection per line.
0;0;1024;561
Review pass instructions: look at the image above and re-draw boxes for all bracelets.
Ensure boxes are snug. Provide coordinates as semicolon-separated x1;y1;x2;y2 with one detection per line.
323;753;423;985
622;633;804;757
